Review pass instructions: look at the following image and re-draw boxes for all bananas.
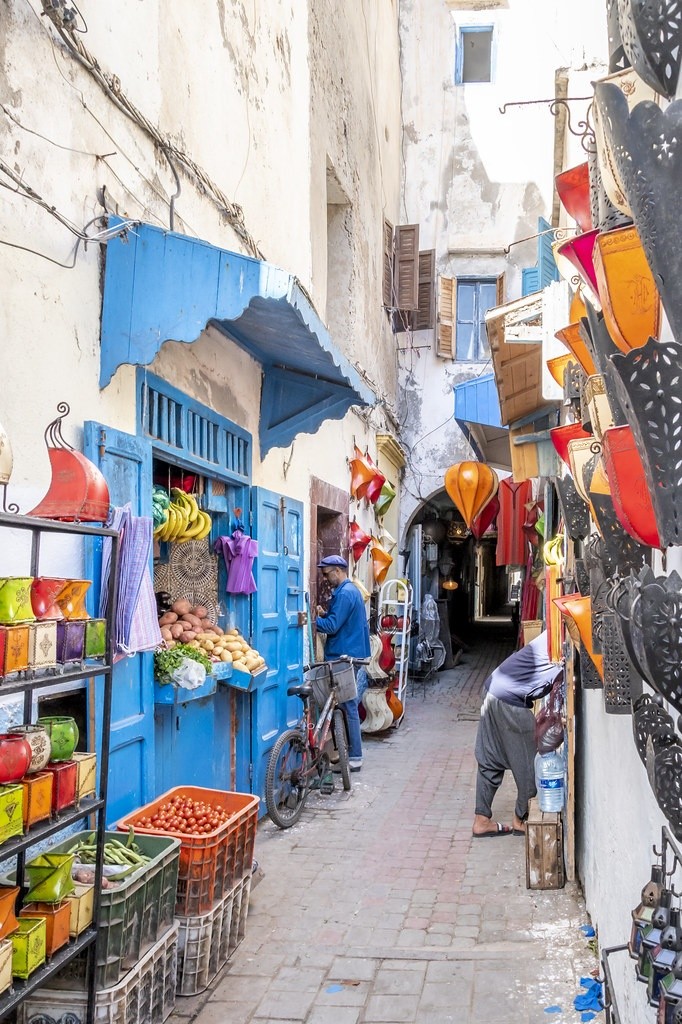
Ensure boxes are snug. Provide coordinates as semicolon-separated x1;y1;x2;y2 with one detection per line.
152;487;211;545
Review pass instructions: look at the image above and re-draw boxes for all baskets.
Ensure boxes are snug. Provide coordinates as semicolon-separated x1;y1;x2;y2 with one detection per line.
305;661;358;706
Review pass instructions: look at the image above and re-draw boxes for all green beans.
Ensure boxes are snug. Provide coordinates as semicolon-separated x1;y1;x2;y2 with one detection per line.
66;823;157;881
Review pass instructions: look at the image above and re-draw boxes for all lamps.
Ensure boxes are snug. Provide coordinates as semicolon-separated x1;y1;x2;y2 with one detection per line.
445;0;682;1024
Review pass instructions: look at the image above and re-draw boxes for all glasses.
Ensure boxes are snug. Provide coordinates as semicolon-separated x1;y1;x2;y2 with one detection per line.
322;567;344;577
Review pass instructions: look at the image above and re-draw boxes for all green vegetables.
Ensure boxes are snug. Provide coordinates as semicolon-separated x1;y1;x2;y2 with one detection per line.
151;642;213;686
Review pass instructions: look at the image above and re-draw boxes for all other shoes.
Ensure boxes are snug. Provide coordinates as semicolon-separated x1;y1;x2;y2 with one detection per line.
331;759;361;773
330;753;351;765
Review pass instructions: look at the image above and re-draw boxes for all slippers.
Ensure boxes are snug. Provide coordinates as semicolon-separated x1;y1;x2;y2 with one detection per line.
513;828;525;836
473;821;514;838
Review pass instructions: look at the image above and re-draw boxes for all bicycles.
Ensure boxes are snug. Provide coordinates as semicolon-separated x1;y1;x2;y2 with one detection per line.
265;655;371;829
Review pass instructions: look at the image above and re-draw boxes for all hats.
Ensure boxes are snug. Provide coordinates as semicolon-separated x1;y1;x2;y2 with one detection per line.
316;555;348;569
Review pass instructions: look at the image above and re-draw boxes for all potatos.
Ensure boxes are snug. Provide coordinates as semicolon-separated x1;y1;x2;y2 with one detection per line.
158;600;266;675
69;867;120;890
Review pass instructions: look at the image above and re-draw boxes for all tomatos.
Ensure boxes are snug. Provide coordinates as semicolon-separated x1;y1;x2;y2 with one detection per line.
127;795;239;836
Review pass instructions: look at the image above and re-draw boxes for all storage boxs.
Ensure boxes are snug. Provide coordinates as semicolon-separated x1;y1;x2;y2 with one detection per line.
524;798;565;890
0;575;270;1024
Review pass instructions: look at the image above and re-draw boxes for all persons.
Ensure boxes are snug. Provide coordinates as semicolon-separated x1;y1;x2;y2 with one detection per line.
472;629;563;838
314;555;371;773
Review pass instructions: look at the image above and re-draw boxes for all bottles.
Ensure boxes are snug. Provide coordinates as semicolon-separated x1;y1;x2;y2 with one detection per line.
534;750;565;813
0;716;78;786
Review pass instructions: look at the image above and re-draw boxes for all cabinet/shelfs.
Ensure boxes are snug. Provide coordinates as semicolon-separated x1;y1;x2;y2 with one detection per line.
378;579;415;728
0;512;122;1024
